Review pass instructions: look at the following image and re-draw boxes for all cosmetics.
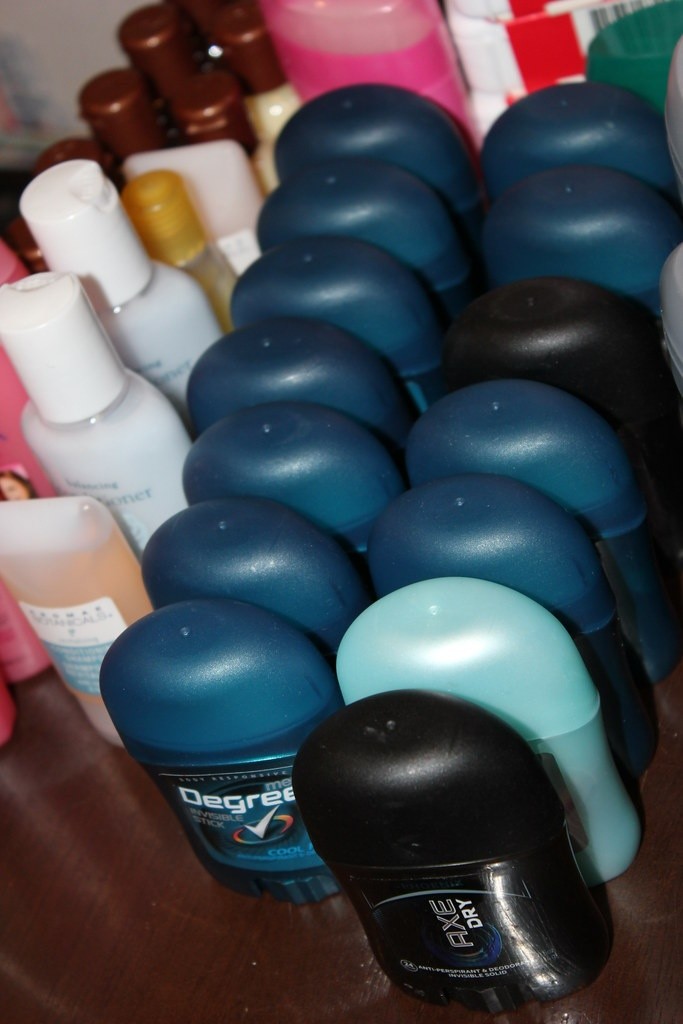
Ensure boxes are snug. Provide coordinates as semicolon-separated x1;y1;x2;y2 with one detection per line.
438;0;607;125
337;577;644;881
478;168;675;325
460;85;657;193
94;608;352;903
294;691;611;1014
371;472;654;777
226;233;444;405
165;399;398;577
251;165;472;334
451;282;681;534
269;88;478;202
260;0;472;113
398;383;675;679
134;497;374;663
183;325;423;485
3;0;294;772
595;5;675;82
